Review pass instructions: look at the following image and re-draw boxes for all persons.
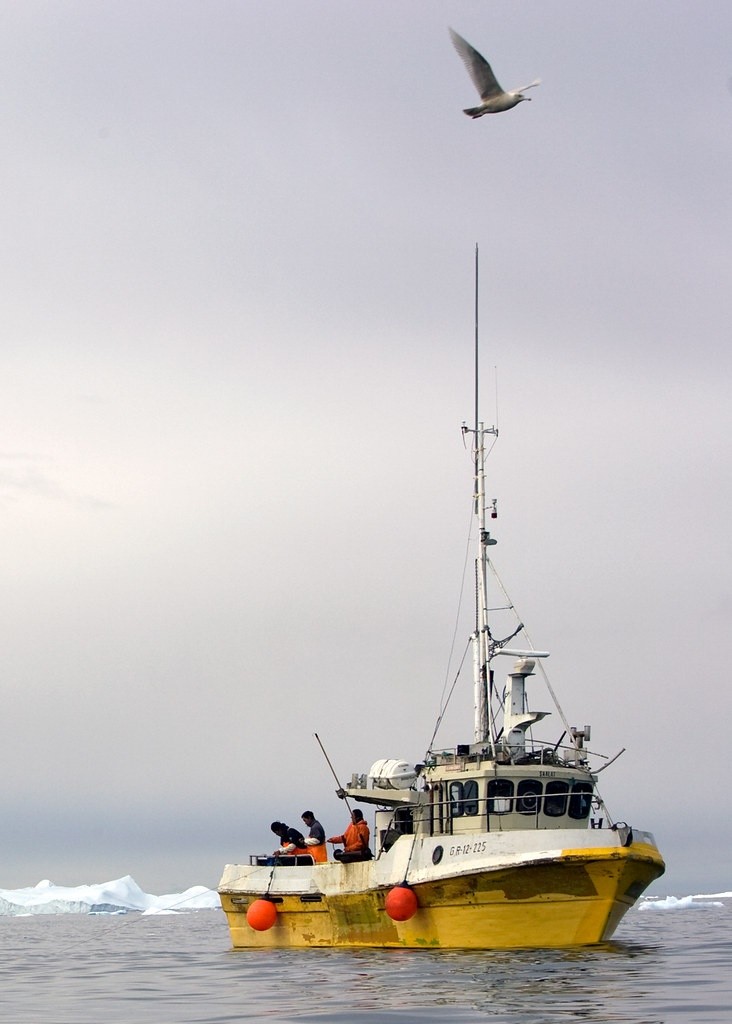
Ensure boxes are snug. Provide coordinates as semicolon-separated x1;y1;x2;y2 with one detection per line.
271;821;307;856
326;809;369;860
301;811;325;846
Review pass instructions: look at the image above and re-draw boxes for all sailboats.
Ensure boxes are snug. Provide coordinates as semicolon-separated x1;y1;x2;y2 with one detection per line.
216;241;666;946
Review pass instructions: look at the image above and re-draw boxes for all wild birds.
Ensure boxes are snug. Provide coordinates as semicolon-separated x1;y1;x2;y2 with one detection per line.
444;22;542;120
